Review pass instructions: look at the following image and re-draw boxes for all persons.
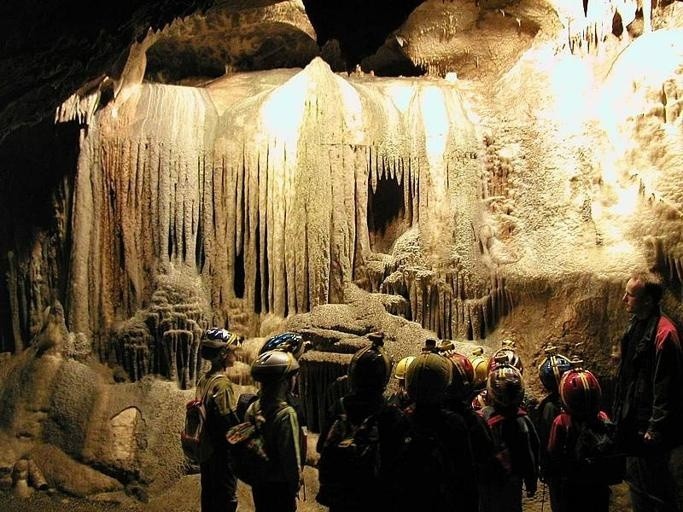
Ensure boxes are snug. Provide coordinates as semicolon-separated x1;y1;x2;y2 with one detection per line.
614;269;683;512
195;326;242;512
242;348;308;511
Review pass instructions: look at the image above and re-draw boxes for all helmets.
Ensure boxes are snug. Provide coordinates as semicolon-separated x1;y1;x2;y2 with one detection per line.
200;327;235;360
407;352;452;402
540;355;572;391
446;354;474;402
257;333;305;360
250;350;299;384
472;360;491;384
559;367;602;418
486;365;526;417
395;356;417;380
347;344;391;390
487;350;523;380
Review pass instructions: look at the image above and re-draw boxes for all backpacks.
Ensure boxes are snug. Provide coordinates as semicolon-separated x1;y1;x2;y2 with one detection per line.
182;374;231;465
319;396;391;511
390;409;465;512
575;434;612;477
486;427;513;485
226;401;289;488
